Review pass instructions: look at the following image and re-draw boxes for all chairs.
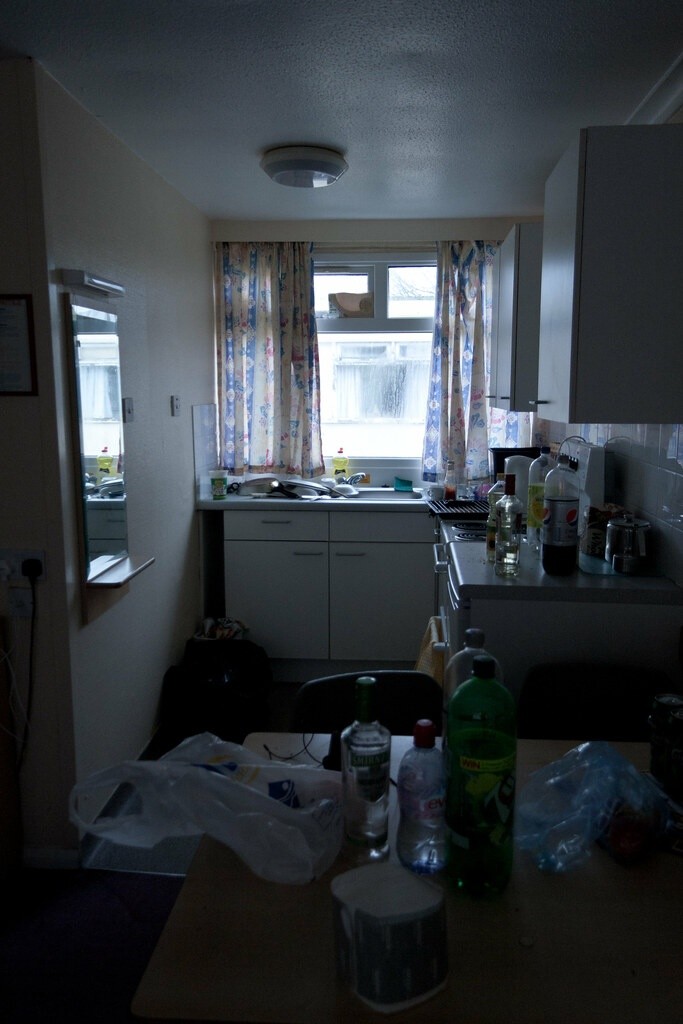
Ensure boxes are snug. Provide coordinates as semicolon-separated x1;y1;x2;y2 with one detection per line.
291;670;443;736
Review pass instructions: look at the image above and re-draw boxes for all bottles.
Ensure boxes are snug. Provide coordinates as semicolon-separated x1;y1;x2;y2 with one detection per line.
396;718;445;875
444;460;457;499
339;676;391;860
542;454;580;575
97;446;113;484
444;627;520;902
527;446;558;550
332;447;349;480
487;473;523;576
605;513;653;573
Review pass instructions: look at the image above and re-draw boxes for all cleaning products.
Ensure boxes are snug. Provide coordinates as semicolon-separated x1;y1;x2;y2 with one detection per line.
331;447;349;480
96;447;114;483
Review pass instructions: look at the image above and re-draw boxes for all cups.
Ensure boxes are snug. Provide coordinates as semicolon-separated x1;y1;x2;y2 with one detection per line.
210;470;228;499
428;487;444;500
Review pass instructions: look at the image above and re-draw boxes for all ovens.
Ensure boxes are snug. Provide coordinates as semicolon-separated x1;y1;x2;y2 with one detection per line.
430;539;472;698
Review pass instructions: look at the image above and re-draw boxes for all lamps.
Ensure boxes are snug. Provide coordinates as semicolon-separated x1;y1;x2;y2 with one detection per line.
259;145;347;187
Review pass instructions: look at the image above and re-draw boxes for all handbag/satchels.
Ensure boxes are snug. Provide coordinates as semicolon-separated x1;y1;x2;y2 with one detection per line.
67;731;346;894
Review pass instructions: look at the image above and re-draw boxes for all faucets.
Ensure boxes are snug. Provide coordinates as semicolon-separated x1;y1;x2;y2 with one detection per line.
341;471;367;486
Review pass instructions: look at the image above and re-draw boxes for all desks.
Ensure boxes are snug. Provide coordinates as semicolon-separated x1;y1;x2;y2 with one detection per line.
127;732;683;1024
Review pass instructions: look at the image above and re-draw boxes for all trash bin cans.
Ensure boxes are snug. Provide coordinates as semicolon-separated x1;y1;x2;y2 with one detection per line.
182;631;264;747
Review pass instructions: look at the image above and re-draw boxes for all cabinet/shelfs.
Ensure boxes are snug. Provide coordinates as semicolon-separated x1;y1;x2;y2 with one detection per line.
86;501;128;554
484;222;543;411
225;510;438;662
433;532;683;740
529;124;682;424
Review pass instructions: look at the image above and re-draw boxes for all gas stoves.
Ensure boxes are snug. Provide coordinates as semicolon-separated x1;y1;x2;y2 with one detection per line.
439;437;606;543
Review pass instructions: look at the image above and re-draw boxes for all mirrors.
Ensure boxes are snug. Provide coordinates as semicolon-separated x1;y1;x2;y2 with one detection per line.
63;292;129;582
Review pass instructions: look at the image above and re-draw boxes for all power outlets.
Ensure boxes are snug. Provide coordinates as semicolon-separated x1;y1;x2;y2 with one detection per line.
172;395;182;416
0;548;47;617
122;398;134;423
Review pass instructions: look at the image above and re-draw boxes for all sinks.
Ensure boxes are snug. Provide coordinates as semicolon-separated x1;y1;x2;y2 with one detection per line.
319;487;431;500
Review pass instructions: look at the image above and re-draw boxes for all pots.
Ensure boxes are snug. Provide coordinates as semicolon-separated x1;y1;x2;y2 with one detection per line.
237;477;331;498
